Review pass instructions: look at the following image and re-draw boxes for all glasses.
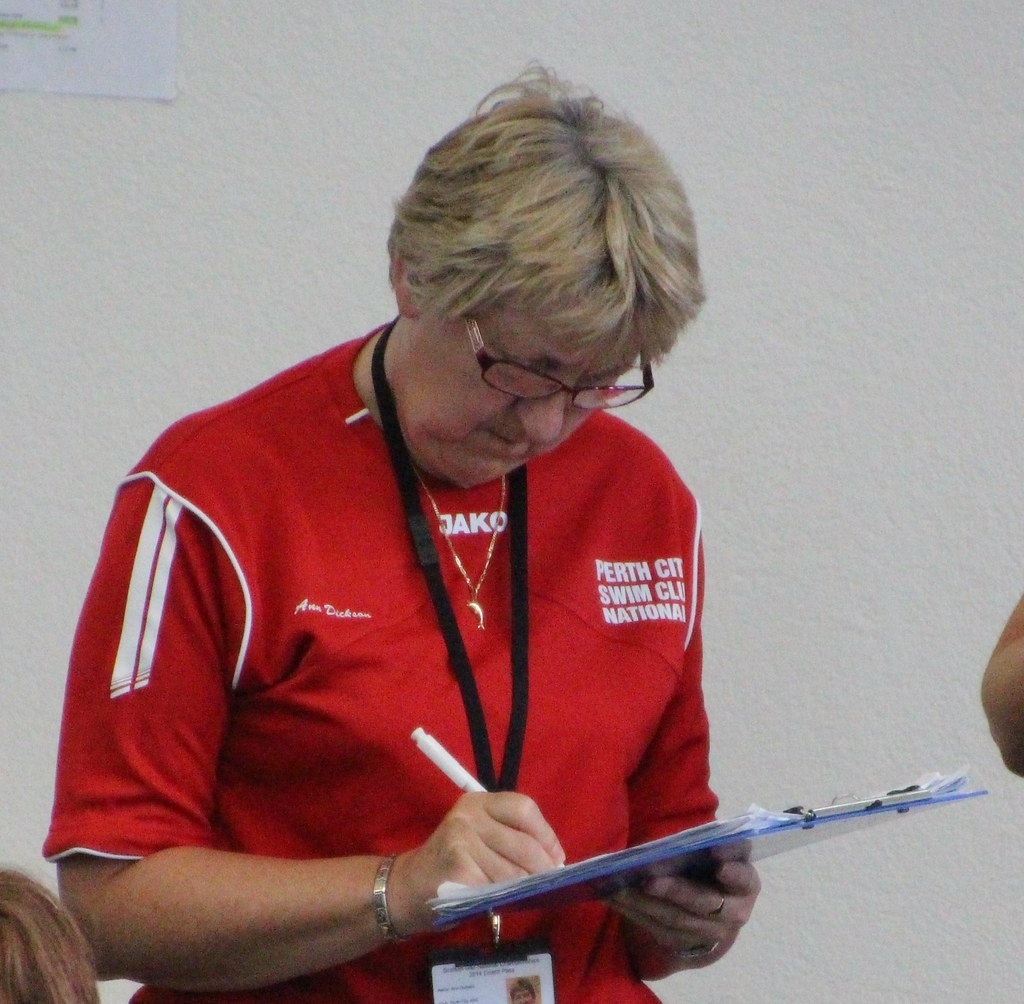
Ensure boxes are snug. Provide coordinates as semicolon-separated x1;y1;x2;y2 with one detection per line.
465;312;653;412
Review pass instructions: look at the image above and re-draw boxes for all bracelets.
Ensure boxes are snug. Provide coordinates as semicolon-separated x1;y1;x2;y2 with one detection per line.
371;853;412;946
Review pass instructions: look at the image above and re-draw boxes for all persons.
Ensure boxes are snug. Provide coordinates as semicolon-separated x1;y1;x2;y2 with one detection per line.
41;74;761;1004
508;977;536;1004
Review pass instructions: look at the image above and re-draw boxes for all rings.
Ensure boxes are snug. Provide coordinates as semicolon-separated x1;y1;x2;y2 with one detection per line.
669;940;722;960
708;891;725;917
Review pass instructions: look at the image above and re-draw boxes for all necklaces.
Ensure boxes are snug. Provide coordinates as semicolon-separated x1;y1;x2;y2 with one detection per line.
408;462;508;631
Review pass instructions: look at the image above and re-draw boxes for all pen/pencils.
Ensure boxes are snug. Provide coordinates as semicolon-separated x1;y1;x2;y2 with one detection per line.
412;728;567;869
